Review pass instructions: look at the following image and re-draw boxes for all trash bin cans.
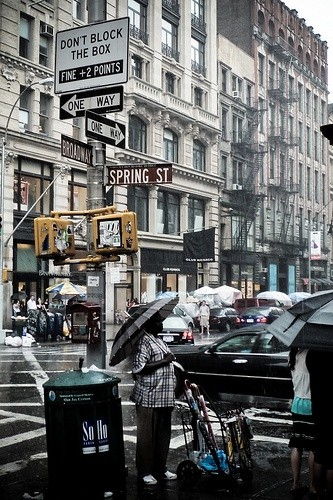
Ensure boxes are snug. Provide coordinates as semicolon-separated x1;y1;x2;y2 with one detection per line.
11;317;29;338
43;370;125;500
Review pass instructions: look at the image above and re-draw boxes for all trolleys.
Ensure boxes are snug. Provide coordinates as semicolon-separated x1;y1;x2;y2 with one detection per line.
174;379;253;494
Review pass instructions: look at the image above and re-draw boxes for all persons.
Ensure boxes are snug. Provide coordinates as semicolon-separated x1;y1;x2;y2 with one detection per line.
12;295;64;317
288;345;318;495
125;298;139;312
305;348;333;495
129;312;178;485
198;300;210;335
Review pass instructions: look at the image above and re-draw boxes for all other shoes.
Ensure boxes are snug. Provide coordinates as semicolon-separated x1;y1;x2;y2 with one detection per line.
153;471;177;480
289;482;316;494
137;474;158;485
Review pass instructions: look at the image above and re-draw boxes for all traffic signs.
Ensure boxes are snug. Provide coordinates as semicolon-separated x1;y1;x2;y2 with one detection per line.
58;85;125;120
85;109;126;150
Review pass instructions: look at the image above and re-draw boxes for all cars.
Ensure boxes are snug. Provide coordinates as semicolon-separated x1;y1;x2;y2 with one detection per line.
236;306;284;329
194;307;239;333
156;314;194;347
167;323;295;399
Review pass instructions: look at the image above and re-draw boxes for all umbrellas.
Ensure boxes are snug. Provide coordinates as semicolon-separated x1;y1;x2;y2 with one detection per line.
45;281;86;308
184;285;242;303
264;289;333;346
108;291;179;367
182;379;254;493
